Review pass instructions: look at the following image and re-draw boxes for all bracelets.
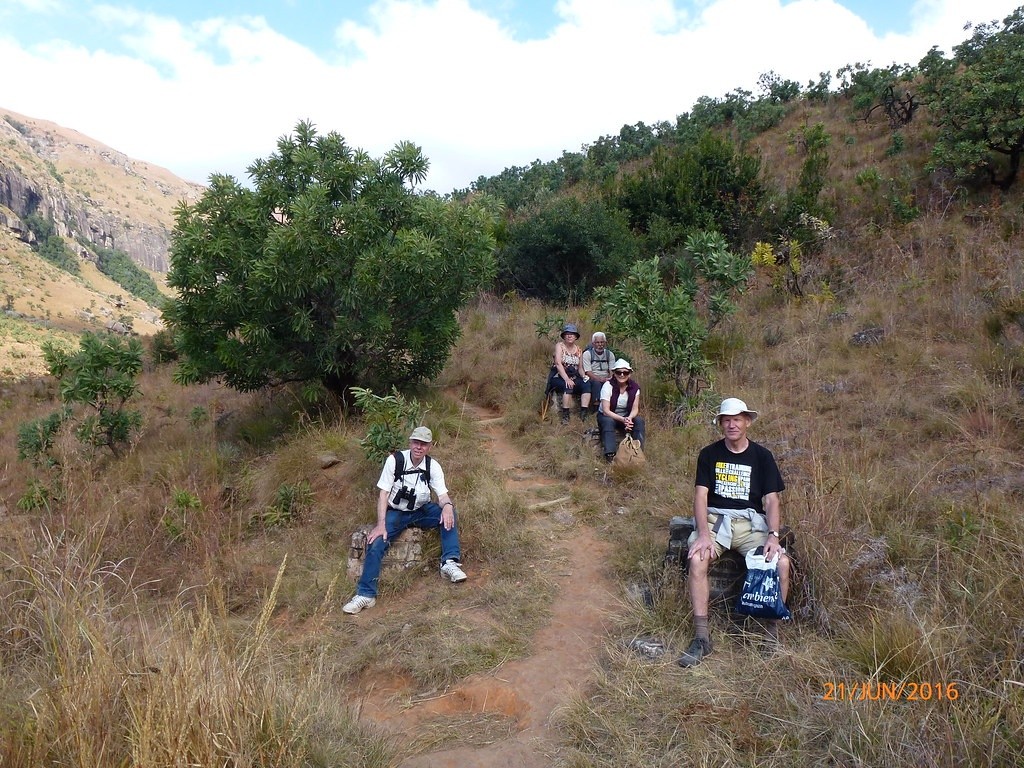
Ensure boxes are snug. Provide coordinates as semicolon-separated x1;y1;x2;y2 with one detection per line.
442;502;454;508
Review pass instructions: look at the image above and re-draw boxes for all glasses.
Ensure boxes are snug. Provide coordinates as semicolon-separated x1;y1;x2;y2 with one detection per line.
615;370;631;375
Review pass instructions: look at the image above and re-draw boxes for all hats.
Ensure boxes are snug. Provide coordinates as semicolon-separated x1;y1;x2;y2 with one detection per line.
560;323;580;339
713;398;757;425
611;358;633;372
409;426;433;443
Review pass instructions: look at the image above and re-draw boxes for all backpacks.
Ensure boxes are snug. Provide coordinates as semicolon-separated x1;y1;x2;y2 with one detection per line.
583;344;609;364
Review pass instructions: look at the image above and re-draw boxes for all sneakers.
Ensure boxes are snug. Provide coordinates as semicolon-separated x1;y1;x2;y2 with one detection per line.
343;595;376;614
758;633;777;657
440;560;467;582
679;636;714;669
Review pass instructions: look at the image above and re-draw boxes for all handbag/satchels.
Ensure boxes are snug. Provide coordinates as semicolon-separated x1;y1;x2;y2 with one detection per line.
734;546;791;620
613;433;645;463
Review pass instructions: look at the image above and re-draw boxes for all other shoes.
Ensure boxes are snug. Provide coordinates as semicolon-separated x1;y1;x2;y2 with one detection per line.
593;406;599;413
606;455;614;464
561;410;570;424
580;409;589;422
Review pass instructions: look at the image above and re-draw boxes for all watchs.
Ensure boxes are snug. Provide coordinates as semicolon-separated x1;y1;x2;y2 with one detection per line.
768;531;781;539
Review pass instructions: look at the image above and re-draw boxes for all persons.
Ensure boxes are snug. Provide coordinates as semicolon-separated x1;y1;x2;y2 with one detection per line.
545;324;592;425
583;332;615;415
597;359;645;465
343;427;467;614
679;398;789;668
